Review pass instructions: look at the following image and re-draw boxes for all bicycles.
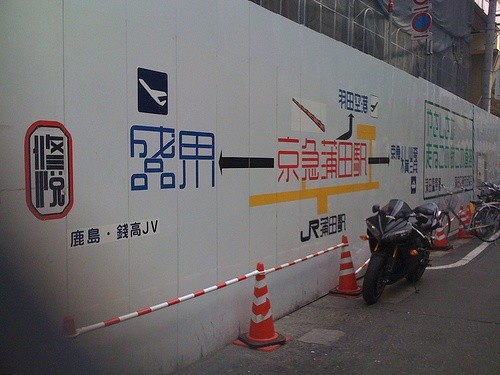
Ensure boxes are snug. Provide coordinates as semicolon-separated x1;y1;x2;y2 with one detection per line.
430;182;500;243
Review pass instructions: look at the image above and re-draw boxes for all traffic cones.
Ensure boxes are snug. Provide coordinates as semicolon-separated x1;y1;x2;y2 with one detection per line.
465;203;472;226
432;224;452;250
329;235;366;296
233;260;292;353
455;205;473;238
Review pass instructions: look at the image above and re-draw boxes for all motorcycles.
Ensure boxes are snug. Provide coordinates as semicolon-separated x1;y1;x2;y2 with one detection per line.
363;199;442;304
